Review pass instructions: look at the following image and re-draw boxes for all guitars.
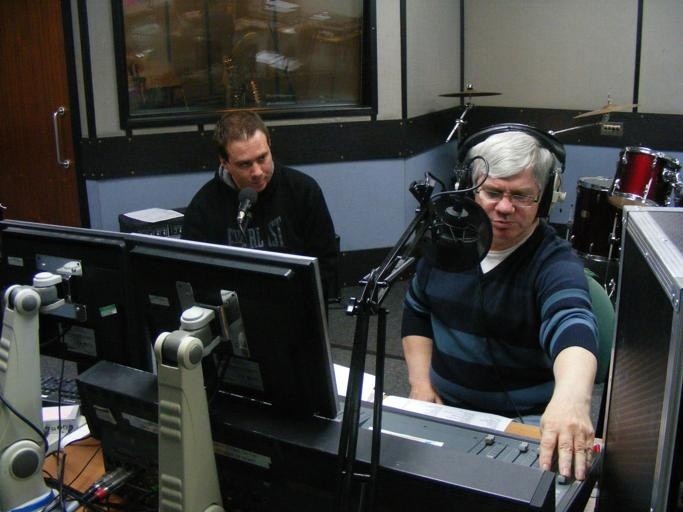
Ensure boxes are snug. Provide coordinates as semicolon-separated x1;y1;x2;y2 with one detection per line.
222;53;238;109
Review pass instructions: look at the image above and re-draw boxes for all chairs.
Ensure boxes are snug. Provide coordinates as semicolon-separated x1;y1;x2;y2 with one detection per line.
585;276;617;385
132;41;224;109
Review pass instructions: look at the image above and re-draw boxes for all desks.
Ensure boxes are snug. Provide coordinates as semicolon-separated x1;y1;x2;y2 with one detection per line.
232;18;361;102
40;422;545;512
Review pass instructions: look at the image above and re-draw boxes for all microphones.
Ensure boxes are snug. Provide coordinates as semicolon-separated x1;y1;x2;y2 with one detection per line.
237;187;258;225
436;167;470;248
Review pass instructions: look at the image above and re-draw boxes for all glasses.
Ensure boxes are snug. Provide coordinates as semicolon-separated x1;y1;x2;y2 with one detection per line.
471;181;546;209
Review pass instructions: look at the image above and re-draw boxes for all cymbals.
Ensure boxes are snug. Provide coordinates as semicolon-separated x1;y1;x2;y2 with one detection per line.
572;104;639;119
437;91;502;96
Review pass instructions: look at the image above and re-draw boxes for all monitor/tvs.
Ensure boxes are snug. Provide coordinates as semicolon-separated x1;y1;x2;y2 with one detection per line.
0;219;153;373
125;232;341;419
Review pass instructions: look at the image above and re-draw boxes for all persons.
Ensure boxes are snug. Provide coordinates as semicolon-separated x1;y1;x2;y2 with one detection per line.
181;106;342;332
399;132;601;484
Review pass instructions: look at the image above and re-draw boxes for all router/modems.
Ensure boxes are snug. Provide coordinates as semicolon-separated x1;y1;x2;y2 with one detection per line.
42;404;80;434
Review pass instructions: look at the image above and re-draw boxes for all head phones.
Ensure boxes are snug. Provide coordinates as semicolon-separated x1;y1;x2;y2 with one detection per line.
448;123;566;218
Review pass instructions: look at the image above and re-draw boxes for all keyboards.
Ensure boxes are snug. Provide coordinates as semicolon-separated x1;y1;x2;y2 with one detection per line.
41;375;80;407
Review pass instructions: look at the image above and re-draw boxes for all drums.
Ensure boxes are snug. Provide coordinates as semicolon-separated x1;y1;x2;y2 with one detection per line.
607;145;682;210
570;176;623;264
